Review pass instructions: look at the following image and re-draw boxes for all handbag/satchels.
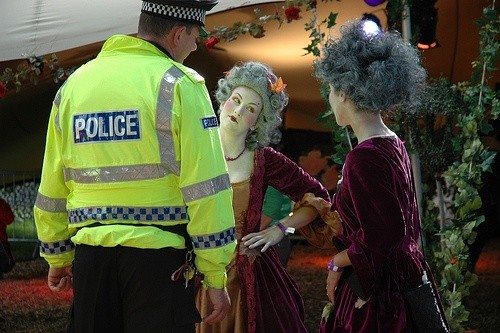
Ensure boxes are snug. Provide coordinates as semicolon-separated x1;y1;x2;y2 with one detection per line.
407;282;449;333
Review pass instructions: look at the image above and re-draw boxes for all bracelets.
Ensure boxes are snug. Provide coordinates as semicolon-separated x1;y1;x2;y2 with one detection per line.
274;222;295;237
328;258;346;272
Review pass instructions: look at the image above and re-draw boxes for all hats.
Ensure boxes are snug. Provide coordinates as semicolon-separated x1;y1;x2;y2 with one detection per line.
140;0;219;38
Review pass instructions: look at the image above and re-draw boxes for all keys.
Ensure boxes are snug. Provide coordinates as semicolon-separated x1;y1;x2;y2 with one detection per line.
172;249;199;288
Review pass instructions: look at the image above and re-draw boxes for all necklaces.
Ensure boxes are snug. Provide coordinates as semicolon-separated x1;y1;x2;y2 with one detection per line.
225;142;247;161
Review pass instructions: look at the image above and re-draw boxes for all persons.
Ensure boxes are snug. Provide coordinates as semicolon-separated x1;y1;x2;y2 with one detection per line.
194;62;329;333
33;1;237;333
311;19;450;333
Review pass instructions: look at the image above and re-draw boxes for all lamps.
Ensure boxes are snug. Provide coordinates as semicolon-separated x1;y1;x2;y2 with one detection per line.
363;7;388;36
